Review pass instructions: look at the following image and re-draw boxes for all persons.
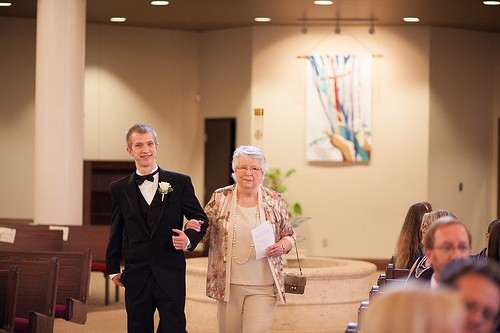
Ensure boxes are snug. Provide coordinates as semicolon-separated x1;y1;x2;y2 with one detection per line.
359;201;500;333
185;145;297;333
104;124;209;333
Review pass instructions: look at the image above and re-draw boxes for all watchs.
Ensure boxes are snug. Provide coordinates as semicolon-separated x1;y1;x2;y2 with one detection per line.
278;242;286;255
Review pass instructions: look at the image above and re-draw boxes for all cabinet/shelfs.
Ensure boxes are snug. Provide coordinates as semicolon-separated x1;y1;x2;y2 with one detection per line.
84;160;135;226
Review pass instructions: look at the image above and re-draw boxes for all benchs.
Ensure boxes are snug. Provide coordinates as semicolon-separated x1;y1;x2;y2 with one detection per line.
0;218;66;251
0;256;61;333
31;226;120;306
0;265;16;333
0;247;93;333
343;254;413;333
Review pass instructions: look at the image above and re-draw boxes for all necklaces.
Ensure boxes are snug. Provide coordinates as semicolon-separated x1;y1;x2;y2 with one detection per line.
233;193;259;264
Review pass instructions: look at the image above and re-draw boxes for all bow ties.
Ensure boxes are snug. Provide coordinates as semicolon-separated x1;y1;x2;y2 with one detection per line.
134;168;160;186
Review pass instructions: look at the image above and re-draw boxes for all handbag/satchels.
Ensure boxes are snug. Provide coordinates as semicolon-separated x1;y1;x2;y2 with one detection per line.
283;236;306;294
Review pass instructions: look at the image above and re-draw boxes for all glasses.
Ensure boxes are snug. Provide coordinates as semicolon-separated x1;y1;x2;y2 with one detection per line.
238;167;261;174
440;244;468;255
422;225;428;230
